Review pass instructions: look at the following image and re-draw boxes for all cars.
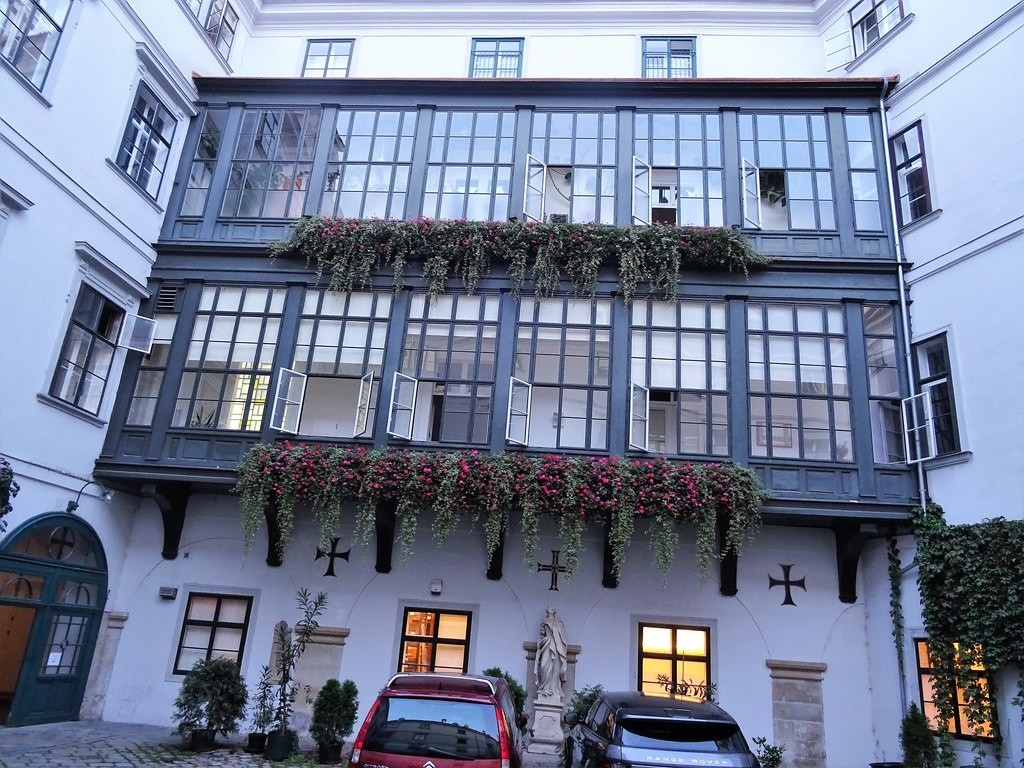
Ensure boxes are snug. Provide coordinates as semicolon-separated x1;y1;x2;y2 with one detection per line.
565;690;761;768
349;670;529;768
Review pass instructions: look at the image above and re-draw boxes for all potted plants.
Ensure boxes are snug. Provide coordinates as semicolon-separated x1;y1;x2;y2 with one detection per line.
266;623;294;762
245;665;274;753
309;678;360;765
168;654;247;752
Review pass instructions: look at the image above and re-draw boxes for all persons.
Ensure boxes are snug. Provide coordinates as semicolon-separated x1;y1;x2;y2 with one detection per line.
534;604;570;698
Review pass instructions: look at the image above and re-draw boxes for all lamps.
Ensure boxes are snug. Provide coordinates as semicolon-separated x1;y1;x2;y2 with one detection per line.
66;480;115;513
